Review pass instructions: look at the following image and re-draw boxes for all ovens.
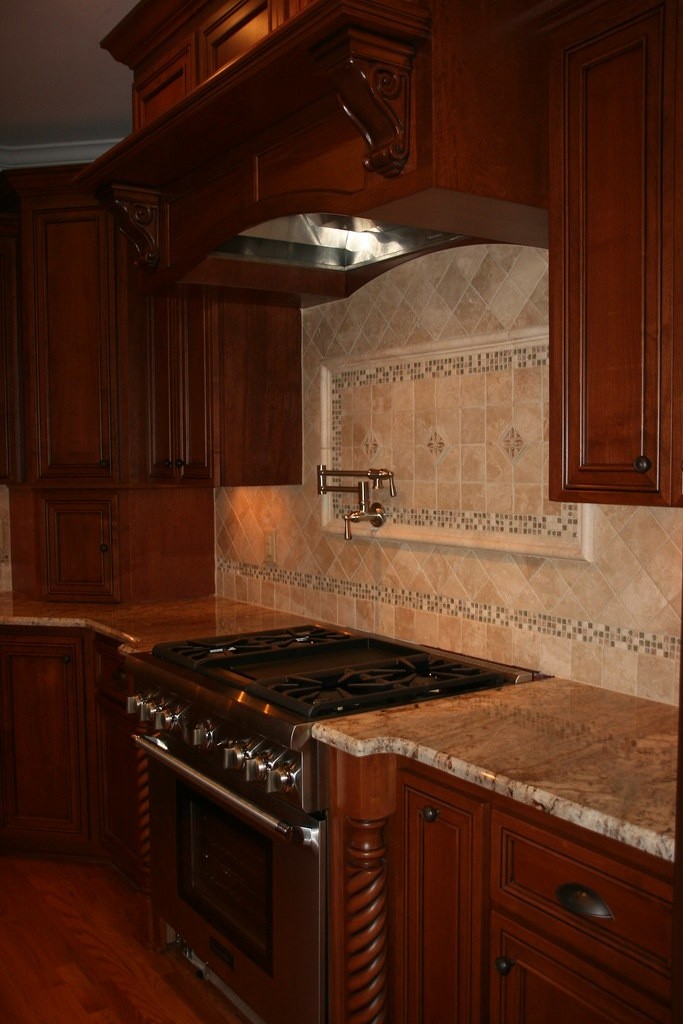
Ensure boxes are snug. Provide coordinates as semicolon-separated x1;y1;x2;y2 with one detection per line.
135;732;325;1024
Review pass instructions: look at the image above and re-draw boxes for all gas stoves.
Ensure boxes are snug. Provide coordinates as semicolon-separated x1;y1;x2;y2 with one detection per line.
136;624;542;823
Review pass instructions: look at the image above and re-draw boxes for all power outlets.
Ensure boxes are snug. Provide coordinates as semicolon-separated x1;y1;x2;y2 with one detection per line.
261;528;276;567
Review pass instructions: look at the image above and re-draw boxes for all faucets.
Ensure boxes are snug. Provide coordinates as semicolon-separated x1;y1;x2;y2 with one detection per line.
340;482;382;541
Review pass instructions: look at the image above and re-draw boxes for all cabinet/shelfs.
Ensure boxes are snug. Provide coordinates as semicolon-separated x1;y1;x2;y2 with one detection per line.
487;790;683;1024
387;755;489;1024
99;0;317;135
5;488;216;604
92;631;147;893
0;624;94;867
547;0;683;507
0;164;303;488
